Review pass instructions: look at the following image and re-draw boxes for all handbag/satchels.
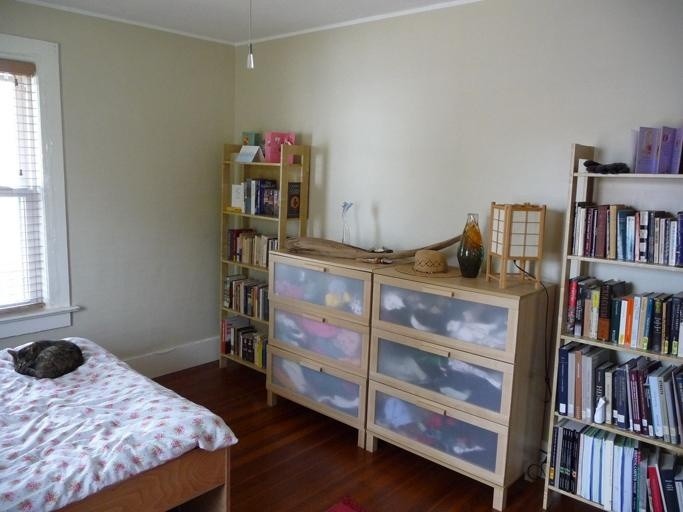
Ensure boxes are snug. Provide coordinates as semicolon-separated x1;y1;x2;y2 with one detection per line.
241;131;296;164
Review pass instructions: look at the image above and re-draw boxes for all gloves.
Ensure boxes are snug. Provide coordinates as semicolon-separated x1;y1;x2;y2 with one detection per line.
583;160;630;174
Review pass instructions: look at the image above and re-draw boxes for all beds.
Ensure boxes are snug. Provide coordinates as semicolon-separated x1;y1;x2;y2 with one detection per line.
0;337;231;512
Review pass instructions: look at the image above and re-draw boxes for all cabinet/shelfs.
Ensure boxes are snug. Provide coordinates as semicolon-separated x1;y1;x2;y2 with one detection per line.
365;261;557;511
266;247;416;450
543;143;682;510
218;141;310;390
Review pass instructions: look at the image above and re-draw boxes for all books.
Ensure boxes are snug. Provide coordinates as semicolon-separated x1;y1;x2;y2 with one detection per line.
550;341;683;512
574;202;682;267
566;277;682;355
633;127;682;173
219;131;300;369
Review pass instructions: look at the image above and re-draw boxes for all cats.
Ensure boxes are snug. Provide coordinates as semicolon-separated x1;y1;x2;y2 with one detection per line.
6;339;85;379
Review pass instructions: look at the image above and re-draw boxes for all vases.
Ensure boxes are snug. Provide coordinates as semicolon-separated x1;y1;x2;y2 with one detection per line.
456;214;485;279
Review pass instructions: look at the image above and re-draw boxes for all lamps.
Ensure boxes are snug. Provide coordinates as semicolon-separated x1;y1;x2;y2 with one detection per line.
485;202;547;291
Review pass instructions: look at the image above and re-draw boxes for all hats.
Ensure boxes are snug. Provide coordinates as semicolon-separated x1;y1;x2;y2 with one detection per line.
394;249;461;278
